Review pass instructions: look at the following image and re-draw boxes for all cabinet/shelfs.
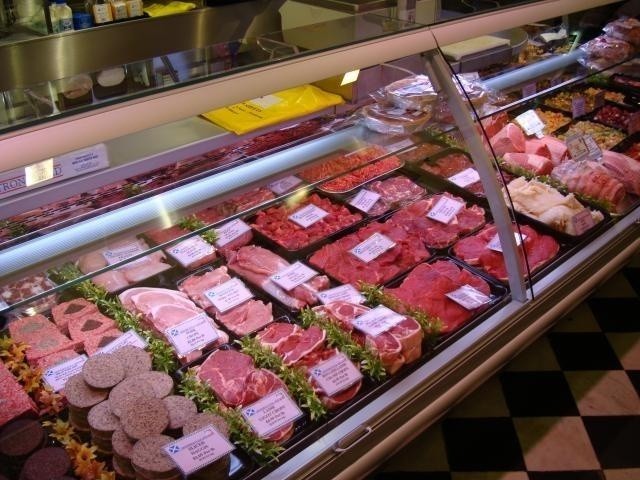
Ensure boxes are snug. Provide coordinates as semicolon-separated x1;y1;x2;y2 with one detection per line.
0;0;640;480
